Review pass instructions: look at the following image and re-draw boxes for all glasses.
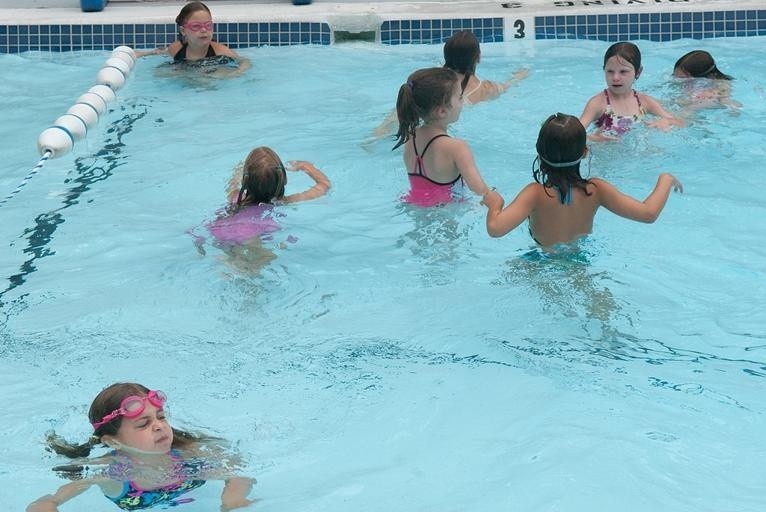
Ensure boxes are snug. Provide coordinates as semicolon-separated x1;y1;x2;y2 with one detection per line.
91;389;166;431
183;20;214;32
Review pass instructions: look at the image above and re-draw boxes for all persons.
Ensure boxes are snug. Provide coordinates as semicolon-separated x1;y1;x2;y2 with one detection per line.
387;68;490;210
478;113;686;249
375;32;532;139
664;50;739;114
21;383;256;510
188;146;332;274
575;41;684;142
134;2;252;78
